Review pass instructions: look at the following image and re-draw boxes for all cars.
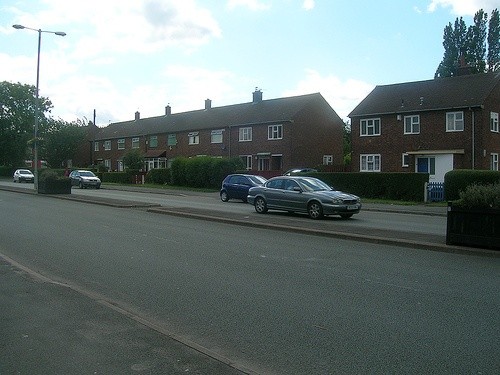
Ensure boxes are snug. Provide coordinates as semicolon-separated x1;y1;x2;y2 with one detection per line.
13;169;34;183
68;170;101;189
219;174;268;203
246;175;361;220
282;167;318;176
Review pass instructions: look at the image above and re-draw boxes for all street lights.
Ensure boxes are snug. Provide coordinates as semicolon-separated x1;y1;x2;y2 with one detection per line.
13;24;67;189
